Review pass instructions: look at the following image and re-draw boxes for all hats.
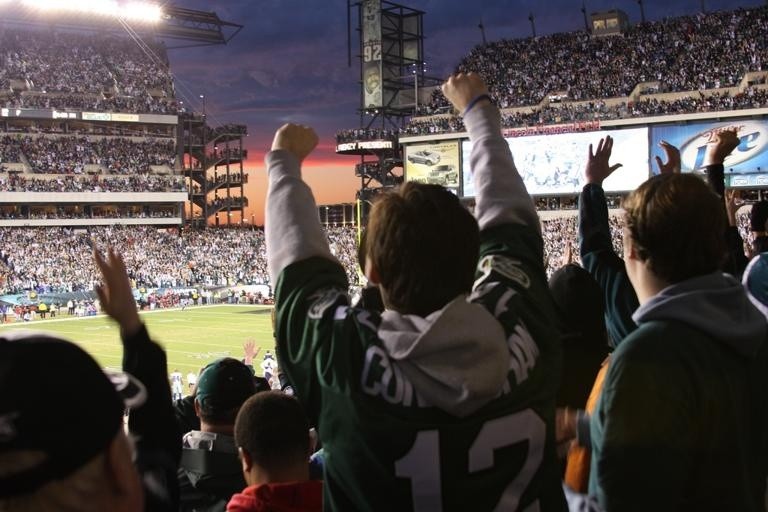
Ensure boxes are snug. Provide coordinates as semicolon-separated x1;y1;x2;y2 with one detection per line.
1;335;125;500
195;357;257;412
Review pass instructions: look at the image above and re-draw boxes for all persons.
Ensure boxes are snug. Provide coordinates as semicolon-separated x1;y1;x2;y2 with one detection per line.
0;0;767;324
0;73;767;512
